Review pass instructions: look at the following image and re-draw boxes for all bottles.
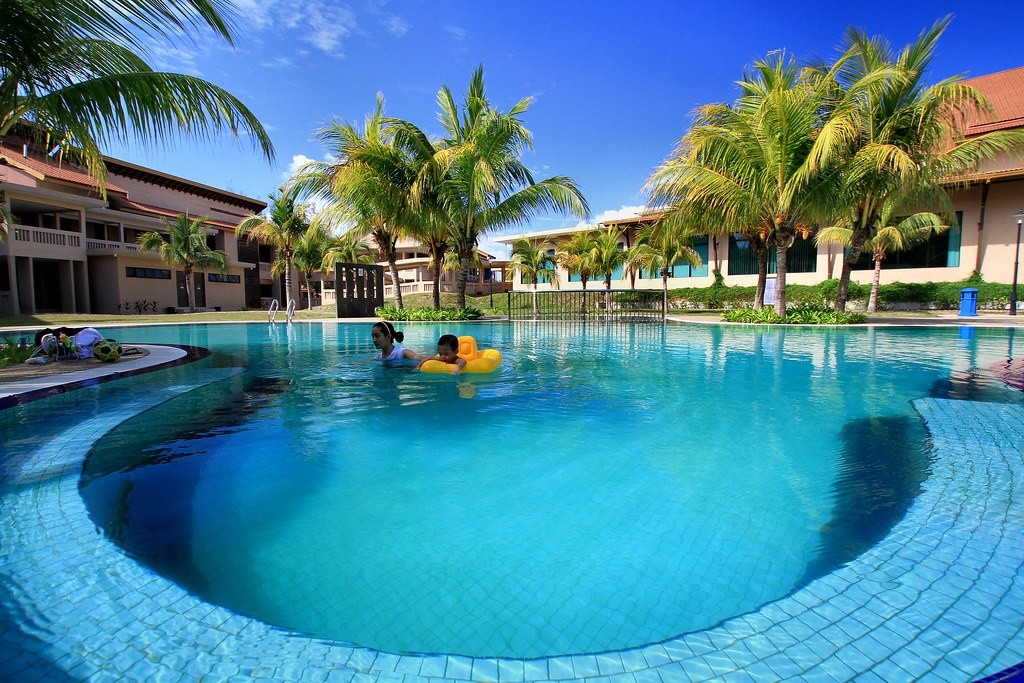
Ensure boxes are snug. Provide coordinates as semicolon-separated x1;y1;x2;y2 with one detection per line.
59;332;75;350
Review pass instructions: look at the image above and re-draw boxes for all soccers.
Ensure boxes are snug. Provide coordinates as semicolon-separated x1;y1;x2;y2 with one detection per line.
93;338;124;363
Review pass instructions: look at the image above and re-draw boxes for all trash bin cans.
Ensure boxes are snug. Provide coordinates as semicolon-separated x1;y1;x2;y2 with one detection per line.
957;288;979;316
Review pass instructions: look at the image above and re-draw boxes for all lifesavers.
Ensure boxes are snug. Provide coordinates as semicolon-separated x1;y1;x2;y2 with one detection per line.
421;335;503;373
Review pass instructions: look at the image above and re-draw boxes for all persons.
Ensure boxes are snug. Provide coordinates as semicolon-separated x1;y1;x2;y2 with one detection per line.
414;335;466;376
371;321;429;362
